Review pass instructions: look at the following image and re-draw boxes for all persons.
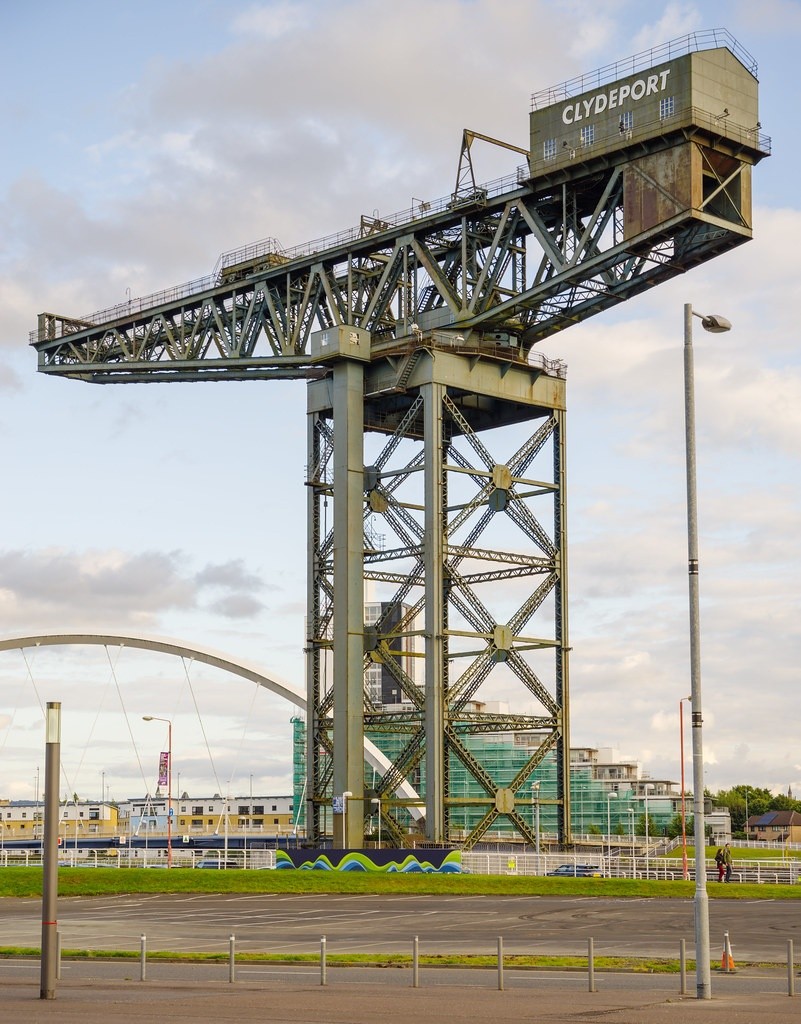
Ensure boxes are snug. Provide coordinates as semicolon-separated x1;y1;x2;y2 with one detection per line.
715;843;733;883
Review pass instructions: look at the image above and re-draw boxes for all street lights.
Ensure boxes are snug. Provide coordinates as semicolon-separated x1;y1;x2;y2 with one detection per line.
607;792;617;878
370;798;381;850
372;766;379;790
681;299;734;1000
143;715;173;869
342;791;353;850
626;808;636;879
745;791;752;847
644;783;655;880
249;774;254;832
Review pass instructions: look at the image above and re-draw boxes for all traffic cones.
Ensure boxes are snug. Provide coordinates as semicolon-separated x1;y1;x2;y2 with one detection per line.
713;929;740;972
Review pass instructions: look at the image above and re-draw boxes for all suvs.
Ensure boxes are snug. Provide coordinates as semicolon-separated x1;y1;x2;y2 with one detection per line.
194;859;239;869
542;863;604;878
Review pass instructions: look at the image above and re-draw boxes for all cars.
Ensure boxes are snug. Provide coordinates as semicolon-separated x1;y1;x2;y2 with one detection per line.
137;863;183;869
58;861;117;868
